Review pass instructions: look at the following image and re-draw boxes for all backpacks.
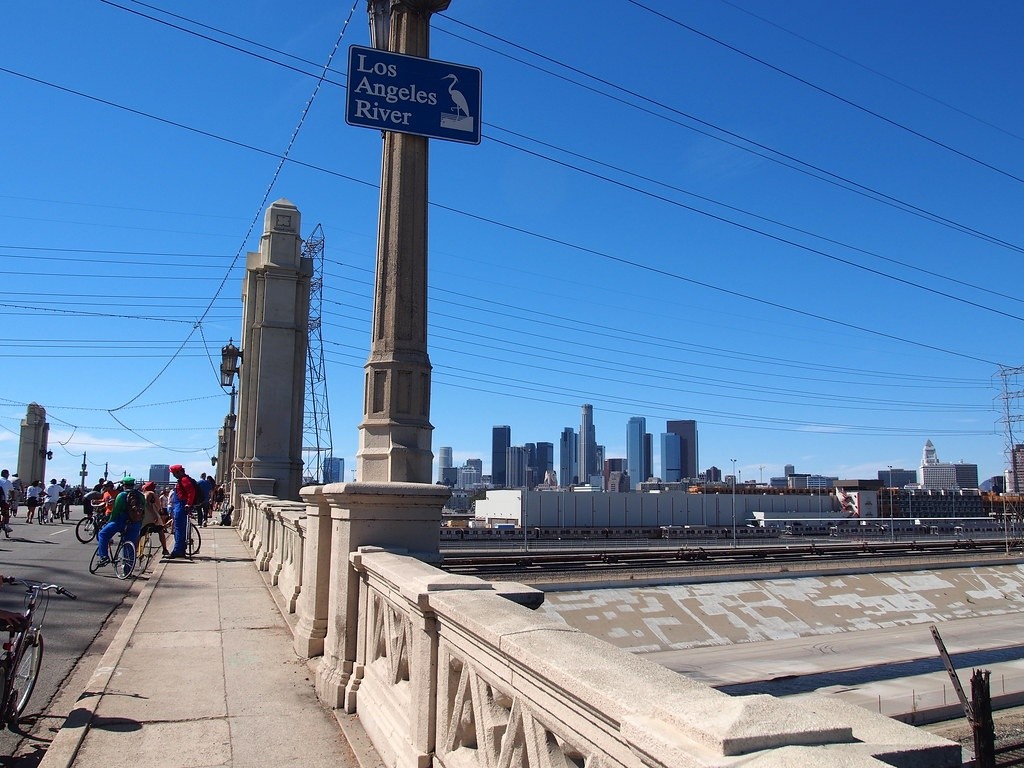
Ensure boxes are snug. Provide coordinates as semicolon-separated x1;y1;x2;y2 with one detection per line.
178;474;205;505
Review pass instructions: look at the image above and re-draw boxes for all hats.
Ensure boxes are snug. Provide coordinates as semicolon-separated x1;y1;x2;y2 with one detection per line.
50;479;56;482
12;474;18;477
121;476;134;484
170;465;183;472
103;480;114;488
99;478;105;482
60;479;66;483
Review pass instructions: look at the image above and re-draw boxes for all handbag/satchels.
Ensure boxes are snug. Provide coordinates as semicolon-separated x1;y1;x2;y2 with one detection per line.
158;507;167;516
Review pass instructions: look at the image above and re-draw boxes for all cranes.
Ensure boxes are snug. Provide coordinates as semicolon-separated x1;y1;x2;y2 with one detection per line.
758;464;766;483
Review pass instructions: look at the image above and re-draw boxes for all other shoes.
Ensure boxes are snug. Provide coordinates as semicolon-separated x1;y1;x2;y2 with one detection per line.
50;518;54;522
9;516;11;518
66;516;69;519
162;549;169;555
97;556;110;565
54;516;57;519
207;515;211;518
14;515;16;517
84;529;90;531
26;518;28;523
122;572;132;578
29;522;33;524
58;517;60;518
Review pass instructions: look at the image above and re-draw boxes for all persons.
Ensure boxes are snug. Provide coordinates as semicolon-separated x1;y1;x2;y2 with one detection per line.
97;476;145;578
10;473;24;516
82;473;225;557
0;469;13;532
73;487;83;503
168;465;200;558
26;478;71;524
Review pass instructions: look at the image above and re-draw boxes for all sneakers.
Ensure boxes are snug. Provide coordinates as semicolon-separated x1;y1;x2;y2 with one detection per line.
203;520;208;527
4;526;13;533
198;524;202;528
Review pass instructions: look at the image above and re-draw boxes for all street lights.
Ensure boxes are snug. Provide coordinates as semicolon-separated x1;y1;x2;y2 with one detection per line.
731;458;737;548
887;466;893;542
220;337;244;386
521;448;531;549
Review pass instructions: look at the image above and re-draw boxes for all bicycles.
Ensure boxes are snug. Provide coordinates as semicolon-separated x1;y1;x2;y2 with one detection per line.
75;500;114;544
1;491;71;536
0;571;77;728
187;505;201;561
137;523;152;574
89;518;137;580
